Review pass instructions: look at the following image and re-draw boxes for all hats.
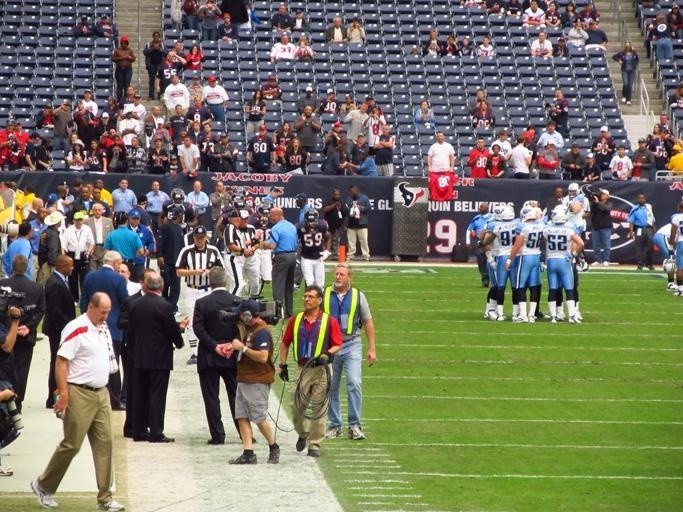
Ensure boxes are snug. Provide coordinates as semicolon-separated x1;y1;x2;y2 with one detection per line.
549;125;646;160
597;188;609;194
7;89;141;148
46;193;84;227
226;186;319;227
119;188;206;235
231;299;259;313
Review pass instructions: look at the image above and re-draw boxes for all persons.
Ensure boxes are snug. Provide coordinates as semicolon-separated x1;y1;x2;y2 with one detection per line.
42;256;76;407
468;182;590;323
592;190;612;266
80;250;190;442
280;265;375;456
194;267;258;443
629;194;683;292
32;291;125;512
1;256;45;475
1;179;377;362
229;301;280;464
0;118;52;172
39;2;683;181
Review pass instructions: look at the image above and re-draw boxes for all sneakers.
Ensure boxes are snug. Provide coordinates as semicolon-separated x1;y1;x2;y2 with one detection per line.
210;425;365;465
96;498;125;512
484;308;583;325
579;261;610;270
666;281;683;296
30;478;58;509
0;466;13;476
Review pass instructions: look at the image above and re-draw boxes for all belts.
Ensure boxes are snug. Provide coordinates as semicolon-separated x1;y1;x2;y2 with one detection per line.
67;382;102;391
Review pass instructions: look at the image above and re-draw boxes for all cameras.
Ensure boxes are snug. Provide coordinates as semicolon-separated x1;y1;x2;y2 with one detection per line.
5;399;24;430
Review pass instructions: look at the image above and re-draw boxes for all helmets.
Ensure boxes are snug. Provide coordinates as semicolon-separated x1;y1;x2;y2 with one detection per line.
493;204;570;225
568;182;579;195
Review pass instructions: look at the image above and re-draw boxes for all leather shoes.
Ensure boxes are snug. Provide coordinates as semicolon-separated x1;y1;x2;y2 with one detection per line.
156;438;174;443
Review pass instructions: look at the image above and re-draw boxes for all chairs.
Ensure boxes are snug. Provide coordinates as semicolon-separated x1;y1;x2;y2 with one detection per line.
2;1;118;178
157;1;637;179
633;1;683;142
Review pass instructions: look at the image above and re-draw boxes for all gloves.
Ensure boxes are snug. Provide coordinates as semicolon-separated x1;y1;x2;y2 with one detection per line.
278;364;290;382
320;250;332;260
316;350;330;366
